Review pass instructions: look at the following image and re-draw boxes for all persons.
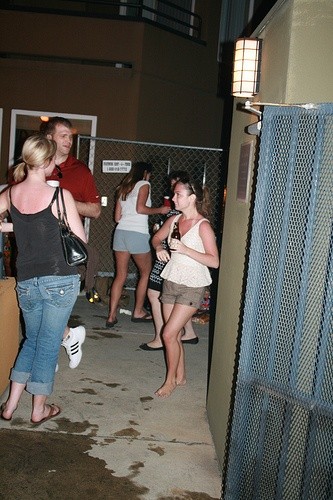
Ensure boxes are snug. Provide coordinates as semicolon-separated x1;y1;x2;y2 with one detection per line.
106;161;172;327
138;171;199;351
7;116;101;372
0;164;18;277
152;175;219;399
0;136;87;425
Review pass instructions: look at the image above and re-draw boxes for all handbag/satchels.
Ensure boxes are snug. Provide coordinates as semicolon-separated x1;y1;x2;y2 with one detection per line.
56;187;88;267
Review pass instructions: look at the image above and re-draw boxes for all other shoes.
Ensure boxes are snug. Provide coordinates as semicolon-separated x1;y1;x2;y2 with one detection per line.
140;344;164;351
182;337;199;344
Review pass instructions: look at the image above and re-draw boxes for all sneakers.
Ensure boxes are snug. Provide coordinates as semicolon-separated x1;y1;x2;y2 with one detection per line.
61;325;86;369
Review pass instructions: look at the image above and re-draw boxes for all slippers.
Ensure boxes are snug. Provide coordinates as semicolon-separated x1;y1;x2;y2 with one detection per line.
31;404;61;424
106;317;117;327
132;311;152;321
1;403;18;421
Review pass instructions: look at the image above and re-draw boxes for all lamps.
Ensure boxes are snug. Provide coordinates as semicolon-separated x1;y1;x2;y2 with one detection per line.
231;38;263;97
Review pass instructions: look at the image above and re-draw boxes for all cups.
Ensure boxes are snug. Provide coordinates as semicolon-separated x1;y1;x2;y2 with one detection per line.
164;196;172;207
46;180;60;188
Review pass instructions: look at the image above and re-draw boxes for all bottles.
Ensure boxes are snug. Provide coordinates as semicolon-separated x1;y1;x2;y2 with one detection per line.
171;222;180;251
